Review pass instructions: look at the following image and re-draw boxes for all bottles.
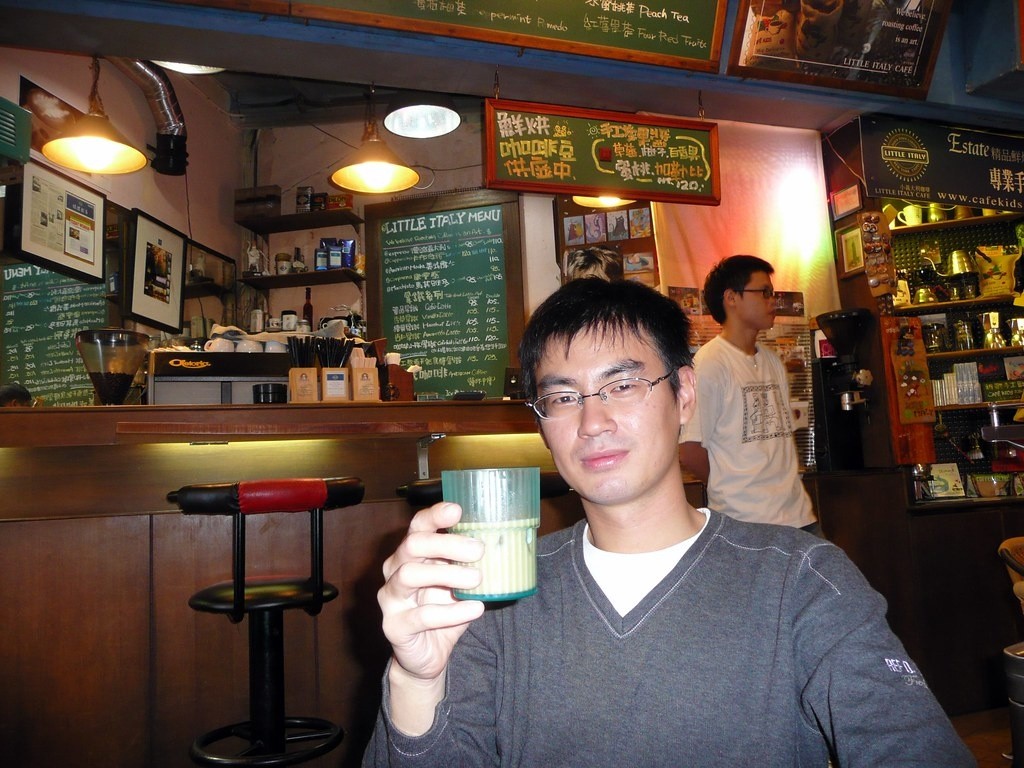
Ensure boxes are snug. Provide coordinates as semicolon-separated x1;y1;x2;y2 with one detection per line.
303;288;314;332
281;311;297;331
351;320;366;338
276;254;291;275
297;320;310;333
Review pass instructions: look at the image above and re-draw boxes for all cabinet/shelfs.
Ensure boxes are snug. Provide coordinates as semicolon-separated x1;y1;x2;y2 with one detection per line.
888;212;1024;716
238;207;365;298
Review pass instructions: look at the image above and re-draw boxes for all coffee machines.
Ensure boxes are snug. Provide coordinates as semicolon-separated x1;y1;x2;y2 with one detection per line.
812;308;877;412
76;328;149;406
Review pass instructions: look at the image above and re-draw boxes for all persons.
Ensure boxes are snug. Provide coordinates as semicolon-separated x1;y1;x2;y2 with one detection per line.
359;277;981;768
997;536;1024;661
680;255;825;540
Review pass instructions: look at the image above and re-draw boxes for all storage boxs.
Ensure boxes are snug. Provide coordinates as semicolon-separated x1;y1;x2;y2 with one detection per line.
320;367;350;402
379;364;414;401
288;367;320;404
350;367;379;400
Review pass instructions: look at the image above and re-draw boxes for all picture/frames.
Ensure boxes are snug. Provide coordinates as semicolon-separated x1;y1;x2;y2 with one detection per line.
829;179;864;222
834;222;867;279
12;157;107;285
123;207;188;334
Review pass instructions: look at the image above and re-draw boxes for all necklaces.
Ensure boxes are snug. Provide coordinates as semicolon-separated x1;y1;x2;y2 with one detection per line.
750;354;758;370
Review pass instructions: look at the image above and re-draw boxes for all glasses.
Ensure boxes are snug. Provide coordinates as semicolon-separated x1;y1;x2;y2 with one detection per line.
525;368;677;420
733;289;774;299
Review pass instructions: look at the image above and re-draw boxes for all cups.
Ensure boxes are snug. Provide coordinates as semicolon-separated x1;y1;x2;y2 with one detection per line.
441;468;541;601
233;340;263;352
893;280;911;307
983;209;997;217
964;282;975;299
949;285;960;301
922;323;945;353
954;322;973;350
323;320;348;329
265;342;287;353
251;383;287;403
897;205;922;226
204;338;234;352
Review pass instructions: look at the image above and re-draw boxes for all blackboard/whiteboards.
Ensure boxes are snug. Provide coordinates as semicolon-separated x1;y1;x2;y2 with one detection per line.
364;190;528;402
485;100;723;206
1;255;109;406
191;0;731;74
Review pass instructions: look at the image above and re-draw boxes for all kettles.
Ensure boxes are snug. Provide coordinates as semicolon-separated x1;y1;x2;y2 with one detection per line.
924;248;991;279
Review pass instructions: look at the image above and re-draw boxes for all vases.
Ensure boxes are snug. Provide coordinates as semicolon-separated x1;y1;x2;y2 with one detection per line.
816;307;871;391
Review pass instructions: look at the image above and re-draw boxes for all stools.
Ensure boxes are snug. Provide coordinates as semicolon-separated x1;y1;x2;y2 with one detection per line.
166;476;368;768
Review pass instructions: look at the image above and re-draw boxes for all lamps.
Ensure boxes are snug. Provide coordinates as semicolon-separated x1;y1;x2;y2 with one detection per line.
39;58;147;176
331;93;420;195
383;89;462;140
